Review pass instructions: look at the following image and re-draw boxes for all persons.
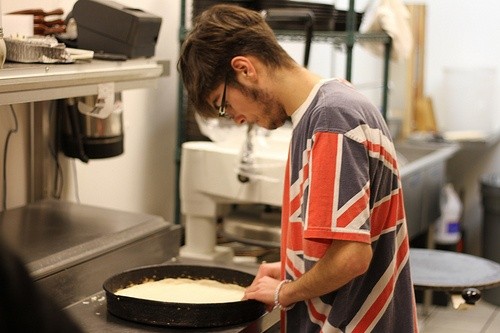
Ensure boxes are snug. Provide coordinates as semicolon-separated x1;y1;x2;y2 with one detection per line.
176;4;418;333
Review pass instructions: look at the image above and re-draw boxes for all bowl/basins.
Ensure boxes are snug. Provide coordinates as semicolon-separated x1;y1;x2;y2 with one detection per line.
335;9;364;31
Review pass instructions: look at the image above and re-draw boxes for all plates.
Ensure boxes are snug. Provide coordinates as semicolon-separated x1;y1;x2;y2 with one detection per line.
103;265;265;328
262;0;335;31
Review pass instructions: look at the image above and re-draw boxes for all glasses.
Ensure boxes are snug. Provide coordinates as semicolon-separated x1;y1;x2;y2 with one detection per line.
216;73;230;121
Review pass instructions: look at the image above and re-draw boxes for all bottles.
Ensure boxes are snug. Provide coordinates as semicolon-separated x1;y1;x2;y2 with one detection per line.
435;183;463;244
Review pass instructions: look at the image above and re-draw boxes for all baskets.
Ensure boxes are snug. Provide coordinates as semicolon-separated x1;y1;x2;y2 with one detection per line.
4;33;66;63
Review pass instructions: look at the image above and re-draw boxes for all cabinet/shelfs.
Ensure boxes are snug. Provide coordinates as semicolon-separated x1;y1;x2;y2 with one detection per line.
0;58;281;333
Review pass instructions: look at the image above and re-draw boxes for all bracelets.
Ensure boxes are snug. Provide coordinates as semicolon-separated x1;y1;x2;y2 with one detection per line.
274;278;296;311
